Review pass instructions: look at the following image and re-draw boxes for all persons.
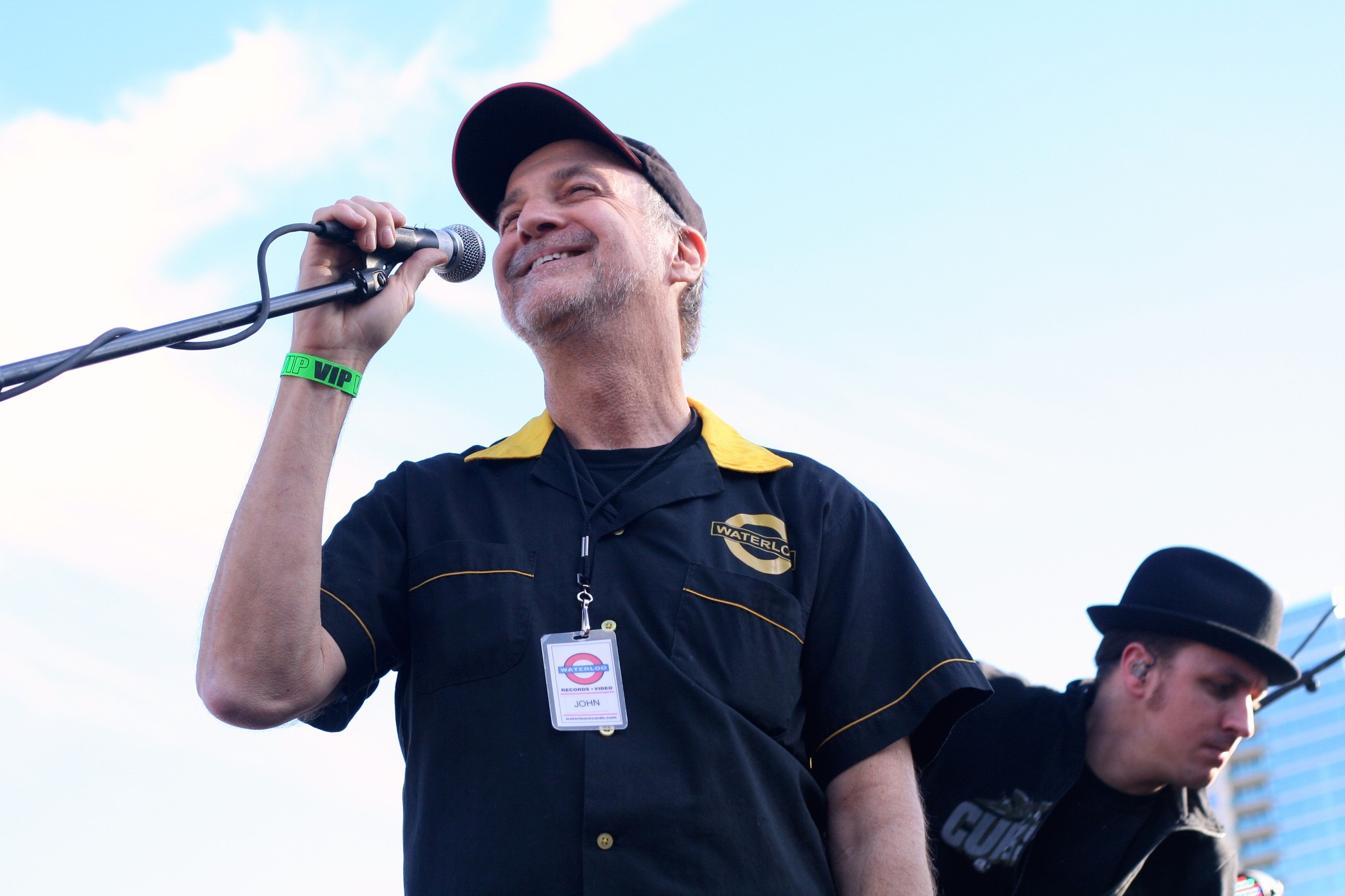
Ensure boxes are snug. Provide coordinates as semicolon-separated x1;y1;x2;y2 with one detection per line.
907;546;1302;896
194;82;994;896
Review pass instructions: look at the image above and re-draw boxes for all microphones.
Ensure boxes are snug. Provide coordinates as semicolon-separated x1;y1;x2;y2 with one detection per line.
315;219;486;282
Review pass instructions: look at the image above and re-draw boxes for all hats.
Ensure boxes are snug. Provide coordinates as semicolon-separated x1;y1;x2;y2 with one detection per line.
451;81;704;239
1090;547;1300;687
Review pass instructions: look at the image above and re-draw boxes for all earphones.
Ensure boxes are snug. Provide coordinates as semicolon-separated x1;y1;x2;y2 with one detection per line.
1131;662;1149;683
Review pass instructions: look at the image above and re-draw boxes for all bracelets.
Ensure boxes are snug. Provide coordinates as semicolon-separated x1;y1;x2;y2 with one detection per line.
281;350;364;399
